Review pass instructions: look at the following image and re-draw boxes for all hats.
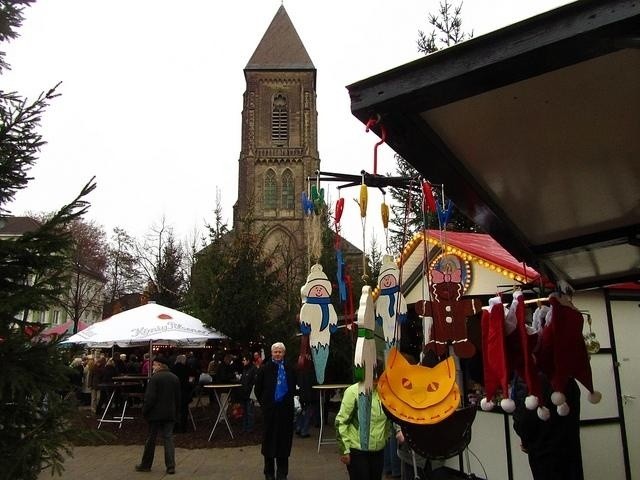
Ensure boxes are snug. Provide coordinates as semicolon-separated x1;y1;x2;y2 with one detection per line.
481;291;602;420
154;357;168;365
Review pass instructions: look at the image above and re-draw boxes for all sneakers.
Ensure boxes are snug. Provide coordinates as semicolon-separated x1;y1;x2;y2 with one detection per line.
135;464;150;472
167;468;175;473
296;430;310;437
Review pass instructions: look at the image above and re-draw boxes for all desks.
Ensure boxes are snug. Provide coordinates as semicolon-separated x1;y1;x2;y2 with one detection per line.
93;376;149;430
312;384;353;454
203;384;243;442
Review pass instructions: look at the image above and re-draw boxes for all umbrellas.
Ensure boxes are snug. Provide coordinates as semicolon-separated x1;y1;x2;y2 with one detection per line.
61;301;232;382
41;320;92;337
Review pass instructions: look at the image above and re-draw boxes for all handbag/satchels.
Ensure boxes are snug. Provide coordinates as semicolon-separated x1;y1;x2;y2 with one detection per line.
378;347;460;423
382;402;476;460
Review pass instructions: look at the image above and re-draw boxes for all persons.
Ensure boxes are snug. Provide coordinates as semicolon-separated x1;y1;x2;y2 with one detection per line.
295;357;315;438
65;341;262;479
334;380;407;479
254;341;298;480
